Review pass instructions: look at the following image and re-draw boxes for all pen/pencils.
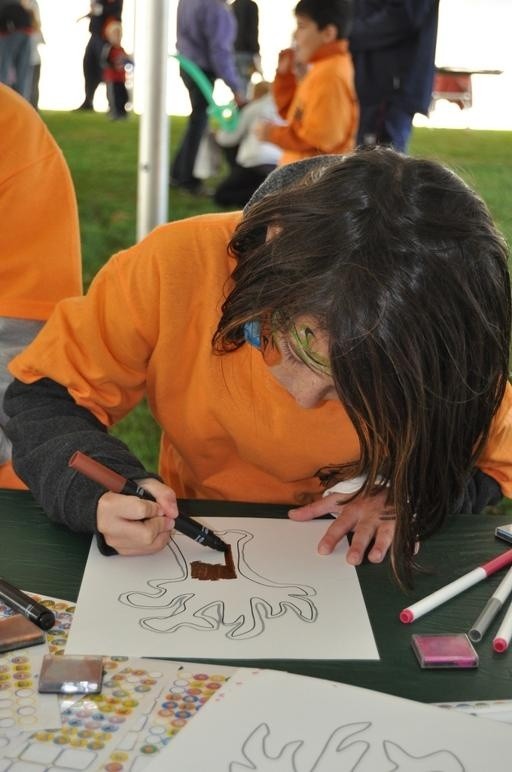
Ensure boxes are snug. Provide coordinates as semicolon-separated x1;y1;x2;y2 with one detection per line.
0;577;56;631
400;548;512;653
68;450;228;551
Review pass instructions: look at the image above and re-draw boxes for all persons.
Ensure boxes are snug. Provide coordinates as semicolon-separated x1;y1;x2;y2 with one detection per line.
96;13;134;124
231;0;262;102
345;0;440;156
254;0;363;172
212;79;286;208
7;145;512;574
167;0;250;193
0;77;87;493
0;0;43;116
70;0;123;114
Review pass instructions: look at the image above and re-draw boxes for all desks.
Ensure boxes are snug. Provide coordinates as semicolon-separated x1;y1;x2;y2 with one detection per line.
0;482;512;768
